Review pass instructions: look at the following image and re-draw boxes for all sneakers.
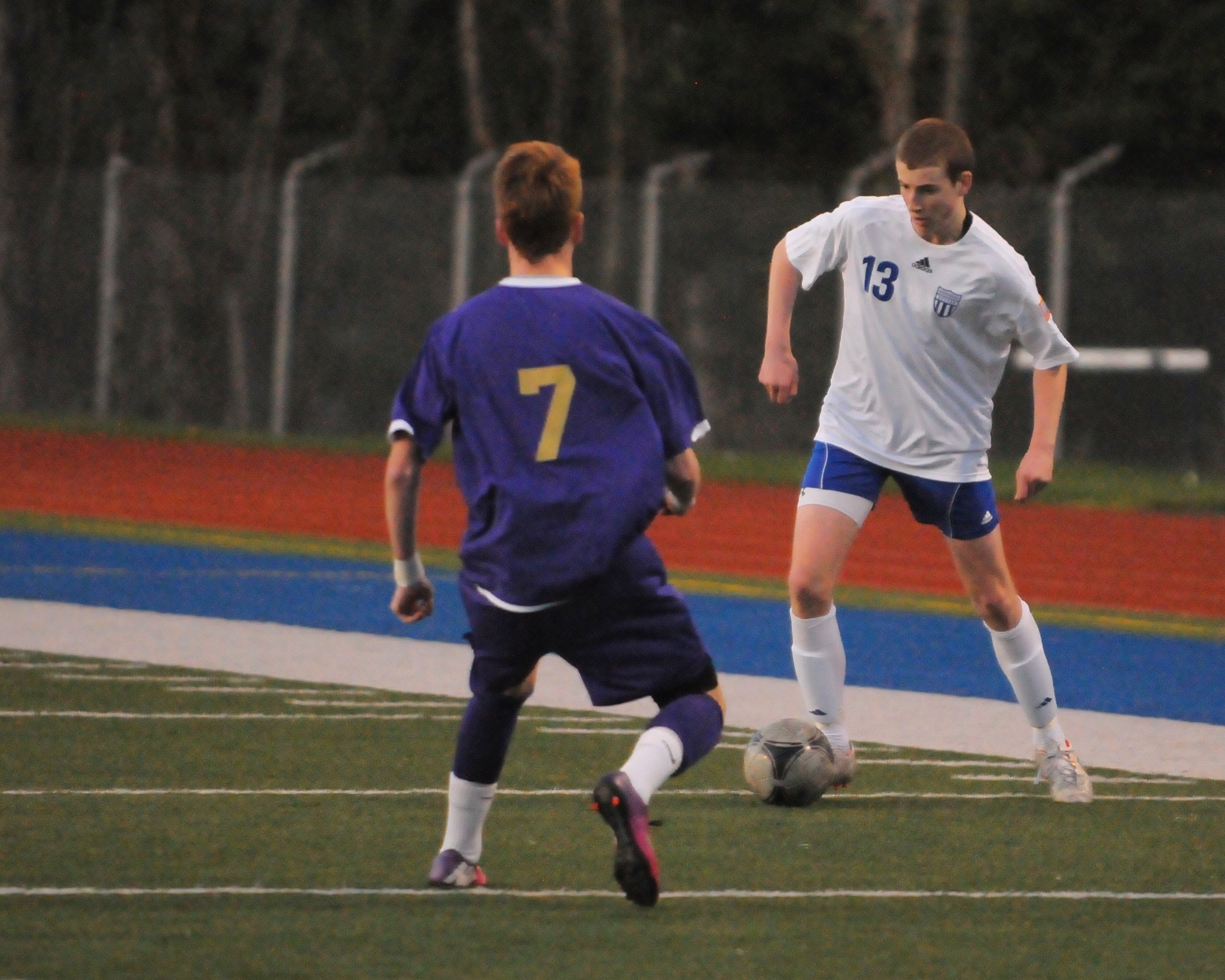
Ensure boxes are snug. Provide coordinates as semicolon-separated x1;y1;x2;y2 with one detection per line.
590;771;663;908
1034;737;1093;804
427;849;486;889
830;742;856;784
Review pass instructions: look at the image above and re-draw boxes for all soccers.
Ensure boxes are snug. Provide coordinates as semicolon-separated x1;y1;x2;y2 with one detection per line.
742;718;833;807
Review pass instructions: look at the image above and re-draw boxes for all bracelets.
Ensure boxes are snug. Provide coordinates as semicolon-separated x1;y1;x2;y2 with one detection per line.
393;552;425;585
668;493;694;513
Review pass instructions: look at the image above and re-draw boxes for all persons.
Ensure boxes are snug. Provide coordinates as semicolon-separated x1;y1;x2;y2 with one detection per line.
386;141;725;908
759;117;1095;803
